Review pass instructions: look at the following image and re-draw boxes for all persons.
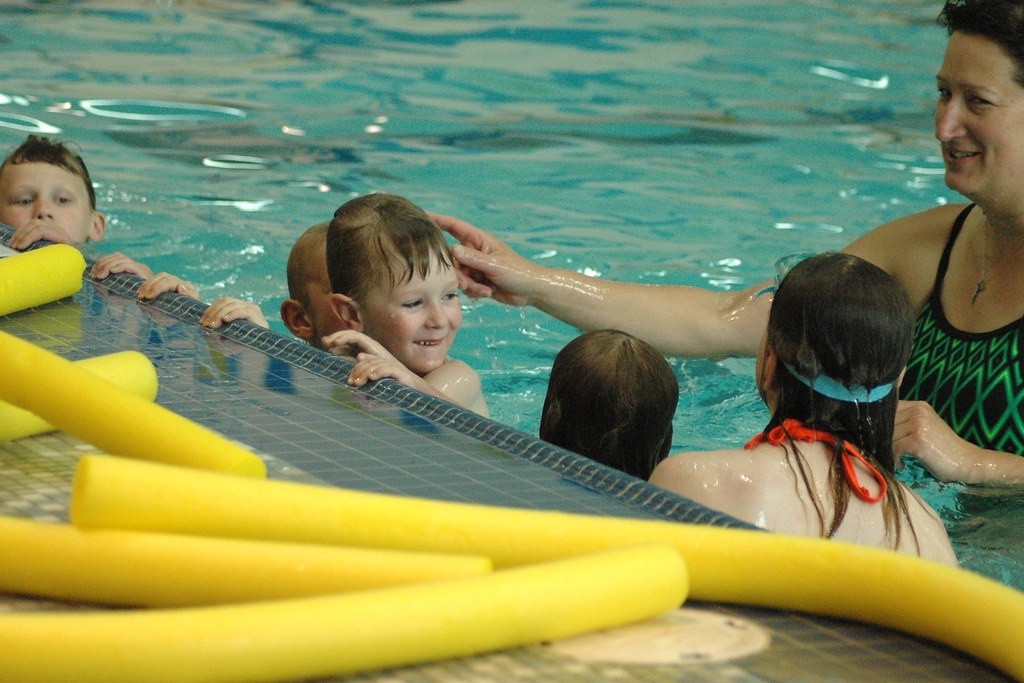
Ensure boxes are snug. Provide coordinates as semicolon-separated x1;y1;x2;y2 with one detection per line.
89;220;347;352
648;253;957;569
539;329;680;481
200;193;480;409
0;135;106;250
426;0;1024;482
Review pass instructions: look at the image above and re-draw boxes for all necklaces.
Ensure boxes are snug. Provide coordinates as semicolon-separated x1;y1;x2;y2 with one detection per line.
970;220;1024;305
